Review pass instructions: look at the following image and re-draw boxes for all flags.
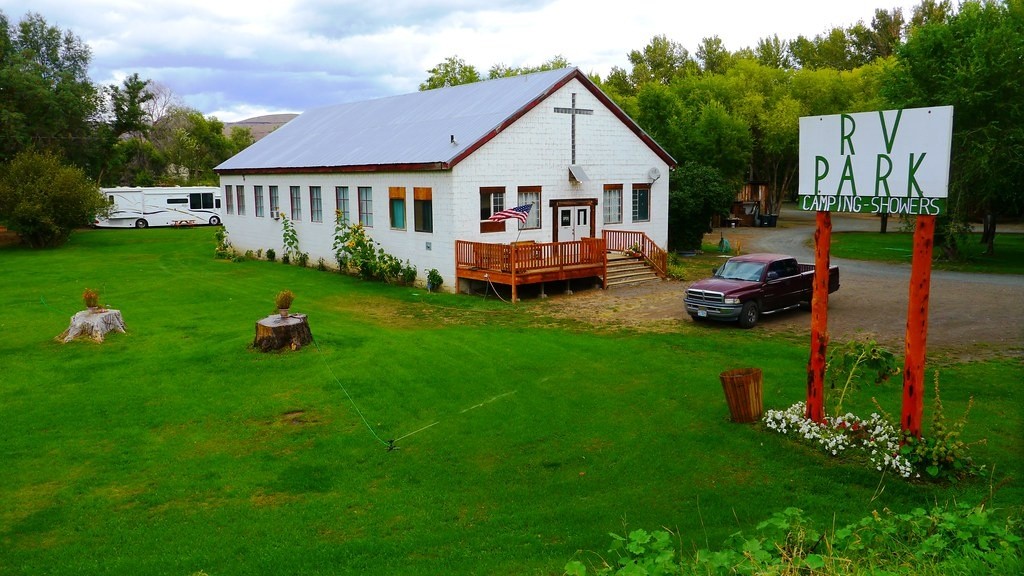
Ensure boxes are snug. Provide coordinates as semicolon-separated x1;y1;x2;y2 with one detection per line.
488;203;533;224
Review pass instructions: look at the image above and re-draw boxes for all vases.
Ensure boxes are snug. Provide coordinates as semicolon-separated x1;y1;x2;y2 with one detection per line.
86;307;98;314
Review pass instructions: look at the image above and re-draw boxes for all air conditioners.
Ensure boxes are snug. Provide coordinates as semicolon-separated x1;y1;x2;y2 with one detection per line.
270;211;279;218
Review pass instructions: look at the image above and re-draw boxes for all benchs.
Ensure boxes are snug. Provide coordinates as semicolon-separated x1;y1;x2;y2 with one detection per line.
476;240;542;265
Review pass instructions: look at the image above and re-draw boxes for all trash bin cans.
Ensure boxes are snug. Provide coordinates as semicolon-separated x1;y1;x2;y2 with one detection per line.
719;368;763;424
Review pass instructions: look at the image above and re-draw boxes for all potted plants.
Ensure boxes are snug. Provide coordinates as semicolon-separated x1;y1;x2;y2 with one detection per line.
275;290;295;318
625;242;641;257
256;249;262;257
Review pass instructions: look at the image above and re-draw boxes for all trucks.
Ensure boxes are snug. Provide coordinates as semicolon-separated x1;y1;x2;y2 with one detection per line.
88;185;221;230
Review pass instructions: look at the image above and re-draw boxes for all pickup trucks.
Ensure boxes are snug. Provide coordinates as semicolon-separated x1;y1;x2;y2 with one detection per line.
681;251;841;329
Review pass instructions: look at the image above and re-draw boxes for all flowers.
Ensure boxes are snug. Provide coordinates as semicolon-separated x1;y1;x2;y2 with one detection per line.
82;288;98;306
